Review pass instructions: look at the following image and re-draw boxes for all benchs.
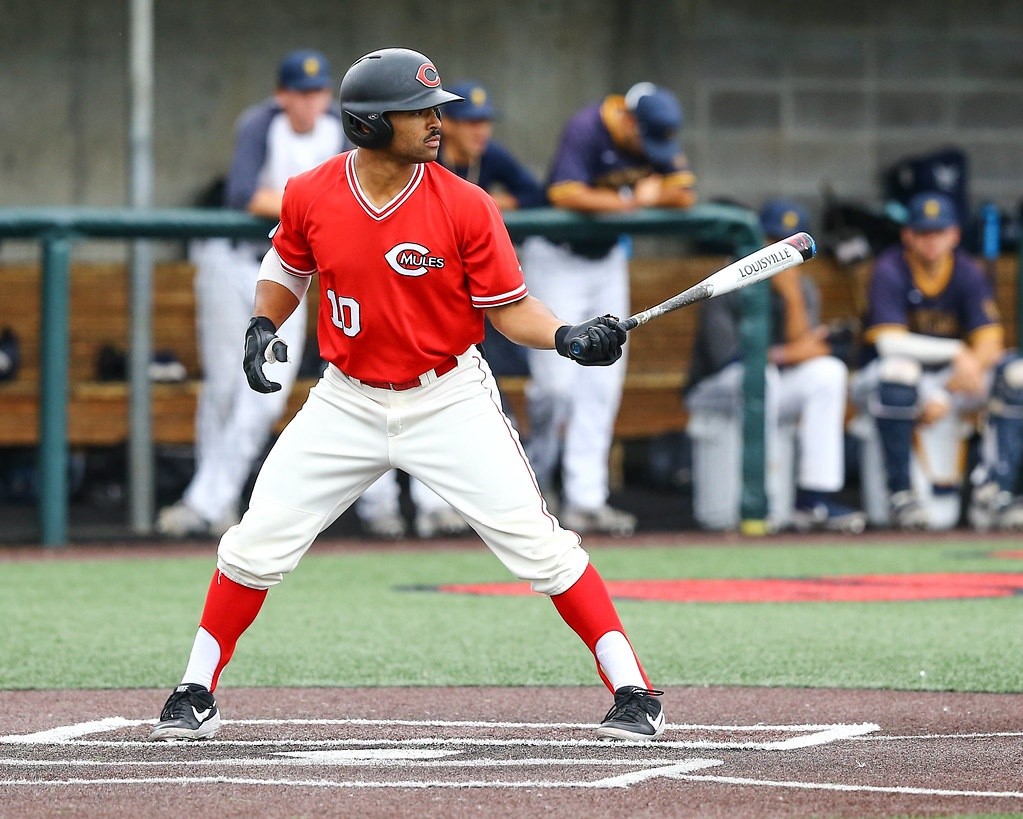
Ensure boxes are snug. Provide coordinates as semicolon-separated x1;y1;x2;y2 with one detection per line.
0;257;1018;445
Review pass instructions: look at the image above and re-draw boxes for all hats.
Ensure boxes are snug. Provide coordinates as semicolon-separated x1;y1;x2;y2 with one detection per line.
760;199;811;242
443;78;496;119
624;80;683;163
909;192;957;233
276;50;330;92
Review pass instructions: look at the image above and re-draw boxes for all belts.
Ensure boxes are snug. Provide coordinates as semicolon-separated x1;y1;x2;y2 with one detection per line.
347;355;458;392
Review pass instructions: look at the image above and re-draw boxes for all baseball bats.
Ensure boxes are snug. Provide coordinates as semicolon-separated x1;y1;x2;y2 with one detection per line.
567;233;819;362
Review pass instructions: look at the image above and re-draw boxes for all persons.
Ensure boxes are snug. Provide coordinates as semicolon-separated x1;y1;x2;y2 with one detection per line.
154;48;712;742
150;49;1023;535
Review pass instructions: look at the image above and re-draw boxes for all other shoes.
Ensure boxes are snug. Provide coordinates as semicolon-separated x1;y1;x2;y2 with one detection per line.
895;493;958;527
365;507;408;537
559;502;639;533
412;508;470;536
798;499;865;532
158;504;243;539
970;496;1023;526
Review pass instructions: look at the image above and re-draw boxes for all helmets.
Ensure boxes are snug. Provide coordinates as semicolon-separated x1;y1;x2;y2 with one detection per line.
340;47;466;149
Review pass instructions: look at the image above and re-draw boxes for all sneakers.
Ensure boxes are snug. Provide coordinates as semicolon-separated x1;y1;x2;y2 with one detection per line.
596;685;665;740
149;683;220;741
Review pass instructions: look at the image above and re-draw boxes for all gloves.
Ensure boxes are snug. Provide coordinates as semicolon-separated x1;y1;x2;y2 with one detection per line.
244;317;288;394
564;314;626;366
